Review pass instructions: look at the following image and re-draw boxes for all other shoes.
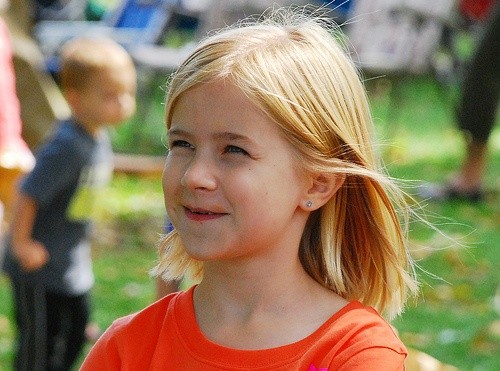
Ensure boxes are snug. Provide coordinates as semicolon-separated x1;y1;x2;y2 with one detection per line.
419;173;481;201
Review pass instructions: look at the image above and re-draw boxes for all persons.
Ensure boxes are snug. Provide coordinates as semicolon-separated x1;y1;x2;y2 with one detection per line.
0;0;187;371
80;6;418;371
323;0;500;214
